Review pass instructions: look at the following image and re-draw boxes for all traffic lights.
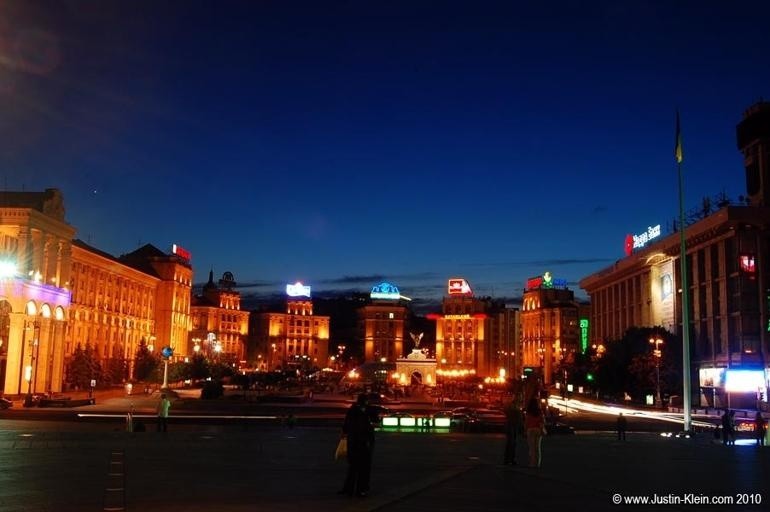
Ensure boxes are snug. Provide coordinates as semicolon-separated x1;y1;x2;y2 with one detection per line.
587;371;591;380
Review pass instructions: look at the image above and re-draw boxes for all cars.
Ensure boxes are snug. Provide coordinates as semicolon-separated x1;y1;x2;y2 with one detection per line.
0;396;13;409
22;390;72;408
387;407;481;428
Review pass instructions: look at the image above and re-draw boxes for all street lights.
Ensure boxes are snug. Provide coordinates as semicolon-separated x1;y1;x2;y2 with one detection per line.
649;337;666;409
20;320;41;407
504;350;515;375
191;338;201;356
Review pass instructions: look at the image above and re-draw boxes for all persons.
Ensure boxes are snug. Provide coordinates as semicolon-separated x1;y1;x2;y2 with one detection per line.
344;403;375;496
617;413;626;441
721;410;730;446
503;401;523;464
524;399;546;467
754;412;765;445
156;395;171;431
712;424;720;444
340;395;368;495
729;410;736;447
